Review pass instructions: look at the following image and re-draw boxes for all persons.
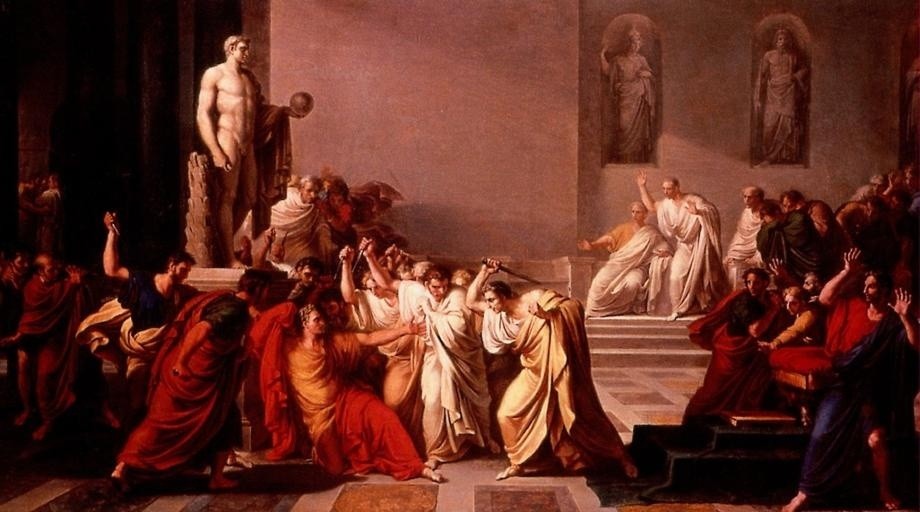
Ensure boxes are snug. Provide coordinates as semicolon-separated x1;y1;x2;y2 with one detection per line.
599;26;658;163
907;55;918;148
752;29;807;165
195;33;308;270
0;167;919;512
